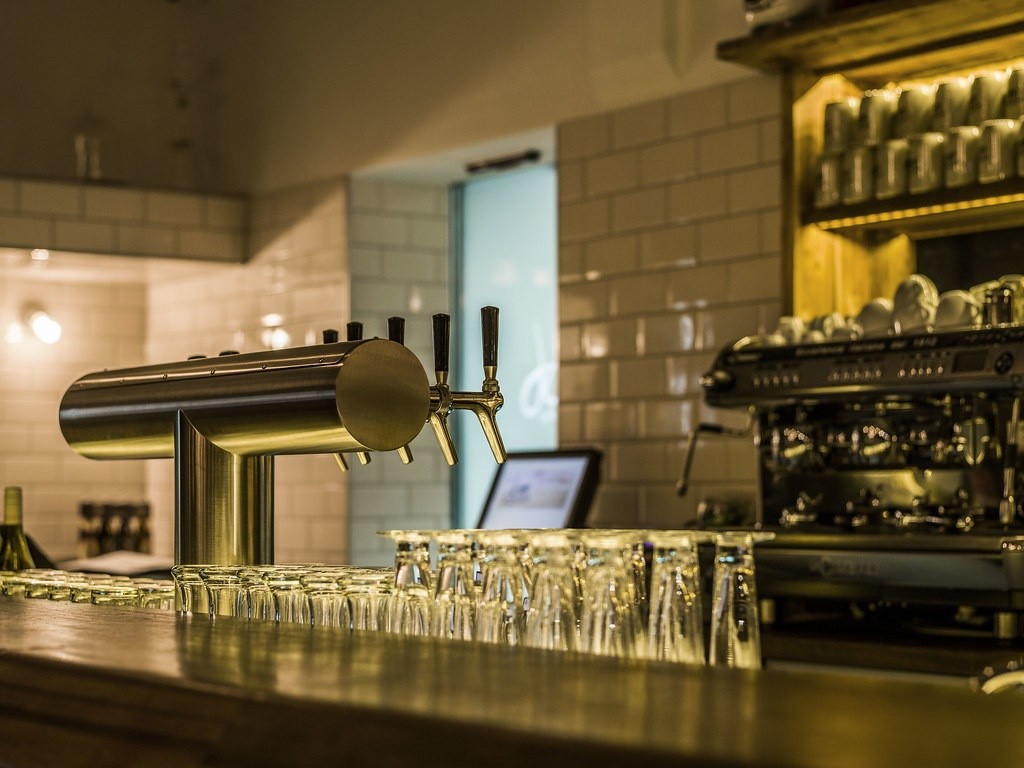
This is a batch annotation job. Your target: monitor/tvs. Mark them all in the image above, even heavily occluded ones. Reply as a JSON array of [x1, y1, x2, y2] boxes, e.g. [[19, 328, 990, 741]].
[[476, 449, 604, 535]]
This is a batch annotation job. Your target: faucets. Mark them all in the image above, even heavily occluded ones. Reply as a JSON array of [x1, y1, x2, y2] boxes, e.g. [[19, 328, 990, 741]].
[[323, 304, 507, 472]]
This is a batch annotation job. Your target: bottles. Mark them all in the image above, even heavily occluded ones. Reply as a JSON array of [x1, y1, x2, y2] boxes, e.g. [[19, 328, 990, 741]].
[[0, 486, 36, 570], [74, 99, 104, 178]]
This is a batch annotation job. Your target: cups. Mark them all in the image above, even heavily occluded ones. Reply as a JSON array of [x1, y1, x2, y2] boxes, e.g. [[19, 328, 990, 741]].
[[0, 567, 176, 611], [522, 528, 580, 652], [470, 530, 532, 649], [761, 274, 1024, 347], [430, 530, 477, 641], [575, 528, 648, 660], [375, 529, 436, 639], [169, 564, 396, 632], [643, 530, 776, 671]]
